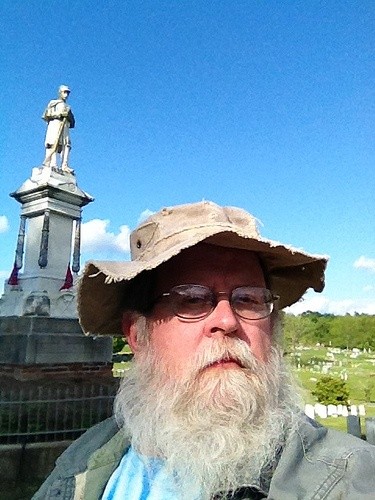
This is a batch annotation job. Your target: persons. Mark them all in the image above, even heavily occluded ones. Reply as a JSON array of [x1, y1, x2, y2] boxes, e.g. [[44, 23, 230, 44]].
[[41, 84, 77, 173], [28, 202, 375, 500]]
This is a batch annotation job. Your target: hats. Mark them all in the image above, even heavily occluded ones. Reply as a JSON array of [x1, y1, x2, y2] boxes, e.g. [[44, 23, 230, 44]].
[[76, 198, 328, 342]]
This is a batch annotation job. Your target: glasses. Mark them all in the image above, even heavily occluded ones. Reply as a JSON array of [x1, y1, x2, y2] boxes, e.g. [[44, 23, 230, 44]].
[[145, 283, 280, 321]]
